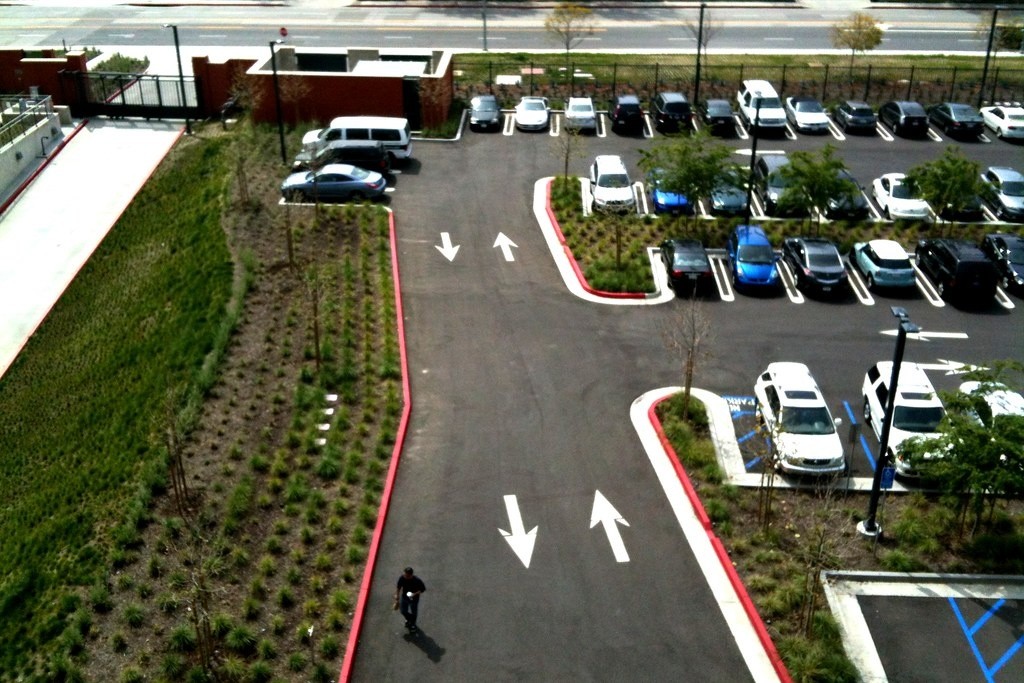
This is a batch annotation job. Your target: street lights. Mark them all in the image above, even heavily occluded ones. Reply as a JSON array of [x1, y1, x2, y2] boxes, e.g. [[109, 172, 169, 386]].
[[160, 24, 194, 136], [857, 303, 921, 538]]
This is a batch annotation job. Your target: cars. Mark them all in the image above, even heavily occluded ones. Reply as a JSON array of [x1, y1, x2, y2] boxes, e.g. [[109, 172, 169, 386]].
[[660, 238, 713, 292], [815, 166, 1024, 221], [785, 95, 829, 135], [834, 100, 1024, 140], [647, 169, 756, 215], [699, 99, 736, 137], [514, 96, 551, 131], [785, 240, 849, 298], [280, 163, 386, 203]]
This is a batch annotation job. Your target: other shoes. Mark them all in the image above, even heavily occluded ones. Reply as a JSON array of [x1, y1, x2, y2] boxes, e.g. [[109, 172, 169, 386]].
[[405, 620, 413, 626]]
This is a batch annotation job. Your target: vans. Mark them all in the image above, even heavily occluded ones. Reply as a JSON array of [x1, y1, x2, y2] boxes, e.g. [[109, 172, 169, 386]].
[[650, 93, 694, 133], [301, 116, 413, 164], [981, 233, 1024, 292]]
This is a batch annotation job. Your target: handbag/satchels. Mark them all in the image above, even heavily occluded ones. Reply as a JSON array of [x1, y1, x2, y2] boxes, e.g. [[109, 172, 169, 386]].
[[393, 599, 399, 611]]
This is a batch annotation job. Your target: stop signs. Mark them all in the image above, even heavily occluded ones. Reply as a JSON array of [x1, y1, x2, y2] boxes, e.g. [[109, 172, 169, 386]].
[[280, 27, 289, 41]]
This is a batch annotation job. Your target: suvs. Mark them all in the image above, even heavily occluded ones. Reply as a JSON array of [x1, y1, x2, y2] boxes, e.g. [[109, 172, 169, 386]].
[[469, 95, 500, 131], [736, 79, 787, 134], [726, 226, 782, 294], [758, 154, 808, 216], [916, 235, 998, 305], [290, 140, 388, 175], [588, 155, 636, 213], [608, 95, 645, 130], [754, 361, 1024, 488], [853, 238, 912, 292], [561, 96, 597, 130]]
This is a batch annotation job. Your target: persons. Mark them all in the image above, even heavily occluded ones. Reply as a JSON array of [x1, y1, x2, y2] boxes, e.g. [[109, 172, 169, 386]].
[[395, 567, 425, 629]]
